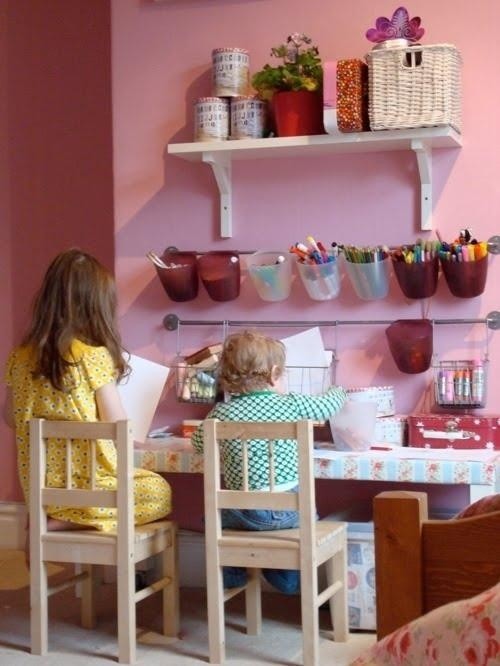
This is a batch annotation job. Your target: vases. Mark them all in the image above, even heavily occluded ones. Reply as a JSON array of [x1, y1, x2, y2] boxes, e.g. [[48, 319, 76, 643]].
[[268, 90, 321, 137]]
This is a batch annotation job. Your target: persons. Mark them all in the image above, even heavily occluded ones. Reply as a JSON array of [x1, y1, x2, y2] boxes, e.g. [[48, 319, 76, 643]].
[[189, 327, 346, 597], [4, 249, 173, 596]]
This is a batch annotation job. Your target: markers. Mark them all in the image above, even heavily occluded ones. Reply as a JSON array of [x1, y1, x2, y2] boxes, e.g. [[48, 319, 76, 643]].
[[440, 357, 483, 404], [228, 256, 237, 266]]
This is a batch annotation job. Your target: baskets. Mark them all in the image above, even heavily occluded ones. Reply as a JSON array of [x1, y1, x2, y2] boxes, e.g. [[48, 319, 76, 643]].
[[169, 356, 219, 404], [364, 44, 462, 134], [282, 349, 338, 429], [431, 352, 486, 408]]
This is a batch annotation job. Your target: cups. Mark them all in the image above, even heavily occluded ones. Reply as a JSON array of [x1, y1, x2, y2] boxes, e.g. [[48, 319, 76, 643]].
[[244, 249, 293, 302], [154, 248, 195, 302], [295, 257, 342, 302], [198, 249, 240, 302], [391, 258, 438, 299], [441, 254, 488, 298], [386, 319, 433, 374], [343, 257, 389, 299]]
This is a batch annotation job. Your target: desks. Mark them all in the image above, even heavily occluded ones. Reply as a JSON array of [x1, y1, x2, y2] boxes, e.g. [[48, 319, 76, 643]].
[[134, 436, 500, 505]]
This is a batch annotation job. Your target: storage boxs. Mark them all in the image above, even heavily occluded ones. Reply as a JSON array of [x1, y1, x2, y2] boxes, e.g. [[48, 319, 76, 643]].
[[321, 504, 462, 630], [408, 416, 500, 449]]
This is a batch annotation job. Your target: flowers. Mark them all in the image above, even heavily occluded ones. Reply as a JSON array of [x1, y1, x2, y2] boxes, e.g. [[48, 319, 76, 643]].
[[252, 32, 323, 100]]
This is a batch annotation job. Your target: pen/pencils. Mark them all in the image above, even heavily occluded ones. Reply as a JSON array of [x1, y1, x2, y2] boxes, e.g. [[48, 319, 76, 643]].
[[436, 227, 487, 262], [252, 256, 285, 289], [389, 238, 437, 263], [147, 250, 189, 269], [370, 446, 392, 451], [343, 245, 389, 299], [289, 236, 338, 299]]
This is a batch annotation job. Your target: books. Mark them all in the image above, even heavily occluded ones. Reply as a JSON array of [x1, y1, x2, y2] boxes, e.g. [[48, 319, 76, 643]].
[[185, 342, 224, 365], [186, 354, 220, 379]]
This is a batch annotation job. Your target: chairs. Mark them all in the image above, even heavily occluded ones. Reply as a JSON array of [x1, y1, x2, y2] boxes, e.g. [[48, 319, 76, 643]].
[[204, 418, 349, 666], [373, 491, 500, 642], [30, 417, 180, 664]]
